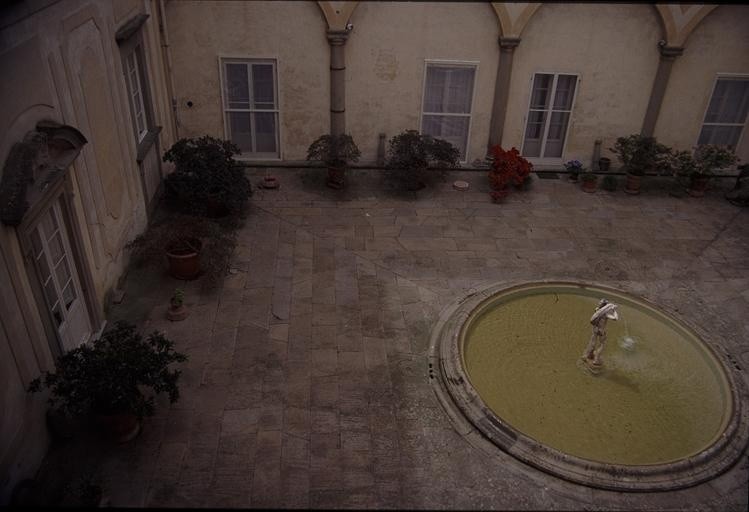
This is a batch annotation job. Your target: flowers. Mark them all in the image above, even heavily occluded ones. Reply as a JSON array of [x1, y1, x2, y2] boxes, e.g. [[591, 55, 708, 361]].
[[563, 159, 583, 172]]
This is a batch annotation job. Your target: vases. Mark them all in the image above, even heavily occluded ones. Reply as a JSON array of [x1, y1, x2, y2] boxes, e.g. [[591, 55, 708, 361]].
[[569, 167, 580, 181]]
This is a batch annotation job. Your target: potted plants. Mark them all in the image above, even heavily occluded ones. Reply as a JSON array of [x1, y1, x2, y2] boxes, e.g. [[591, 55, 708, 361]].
[[581, 132, 748, 207], [27, 322, 188, 447], [166, 290, 190, 323], [122, 211, 235, 279], [305, 130, 532, 204]]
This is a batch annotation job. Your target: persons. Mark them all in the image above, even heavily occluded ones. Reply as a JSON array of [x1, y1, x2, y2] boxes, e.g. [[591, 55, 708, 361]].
[[586, 299, 618, 364]]
